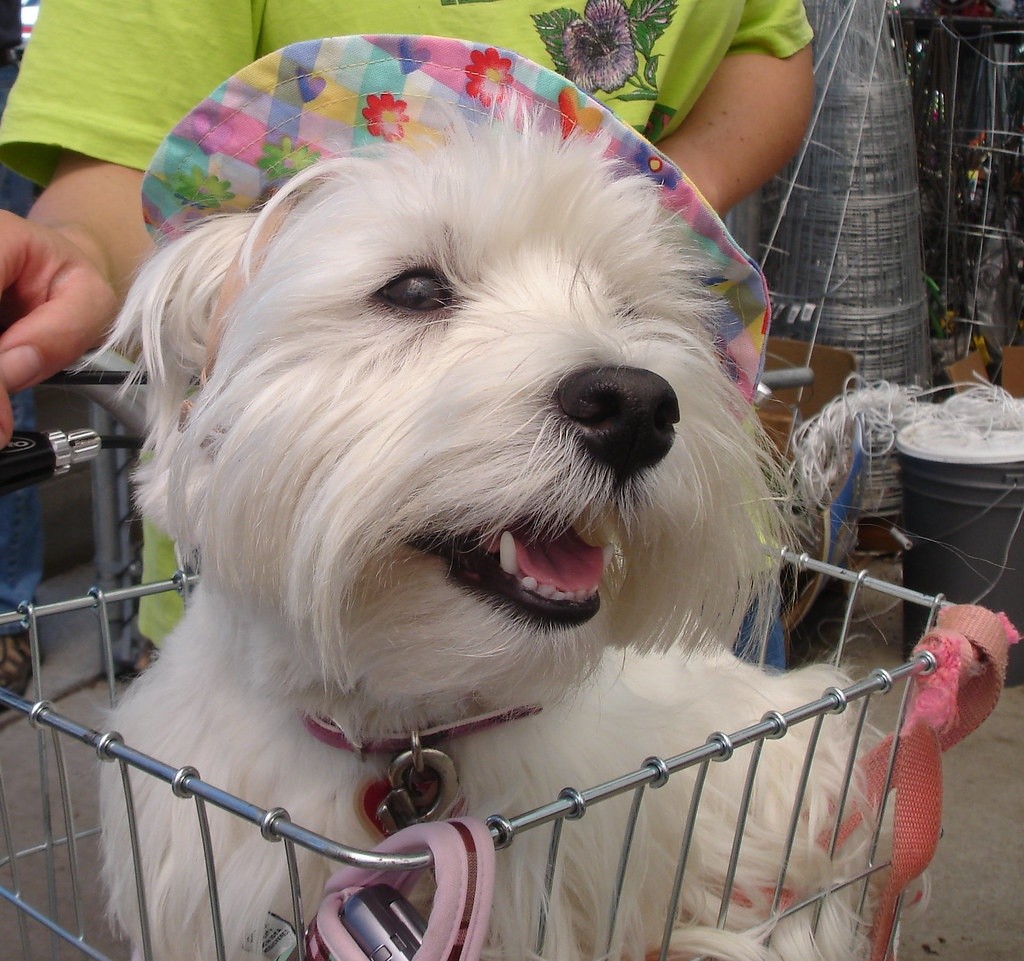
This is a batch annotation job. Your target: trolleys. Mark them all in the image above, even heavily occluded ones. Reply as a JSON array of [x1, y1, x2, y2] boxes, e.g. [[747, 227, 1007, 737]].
[[1, 347, 1019, 960]]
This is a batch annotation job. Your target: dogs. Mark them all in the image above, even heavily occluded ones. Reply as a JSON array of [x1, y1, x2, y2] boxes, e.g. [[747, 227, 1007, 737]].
[[99, 102, 871, 961]]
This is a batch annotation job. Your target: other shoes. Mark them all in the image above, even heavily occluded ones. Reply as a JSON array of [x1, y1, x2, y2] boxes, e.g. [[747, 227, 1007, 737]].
[[0, 628, 32, 711]]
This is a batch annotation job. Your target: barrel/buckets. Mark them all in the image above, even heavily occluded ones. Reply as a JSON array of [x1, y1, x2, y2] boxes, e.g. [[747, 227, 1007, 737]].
[[896, 419, 1023, 683]]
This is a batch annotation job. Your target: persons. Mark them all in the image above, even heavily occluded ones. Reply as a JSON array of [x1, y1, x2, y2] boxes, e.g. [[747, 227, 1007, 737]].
[[0, 0, 816, 670], [0, 64, 46, 713]]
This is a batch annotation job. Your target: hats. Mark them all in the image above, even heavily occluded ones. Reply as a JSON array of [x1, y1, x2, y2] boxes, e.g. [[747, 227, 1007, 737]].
[[144, 34, 771, 423]]
